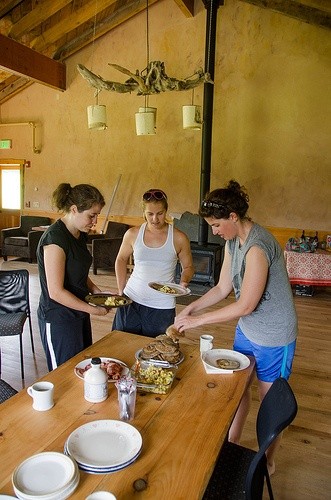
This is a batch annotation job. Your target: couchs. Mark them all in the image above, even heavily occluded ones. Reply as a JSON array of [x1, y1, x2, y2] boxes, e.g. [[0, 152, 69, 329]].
[[0, 215, 51, 264], [86, 221, 132, 275]]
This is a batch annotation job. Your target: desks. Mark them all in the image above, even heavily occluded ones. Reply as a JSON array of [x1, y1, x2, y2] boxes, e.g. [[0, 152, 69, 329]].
[[31, 225, 50, 231], [283, 247, 331, 295], [0, 330, 257, 500]]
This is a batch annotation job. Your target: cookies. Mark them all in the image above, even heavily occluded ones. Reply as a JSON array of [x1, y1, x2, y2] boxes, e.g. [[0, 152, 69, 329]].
[[140, 335, 182, 363]]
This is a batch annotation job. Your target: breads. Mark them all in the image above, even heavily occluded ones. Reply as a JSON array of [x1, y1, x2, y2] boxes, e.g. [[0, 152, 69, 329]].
[[217, 359, 240, 369], [166, 325, 185, 339]]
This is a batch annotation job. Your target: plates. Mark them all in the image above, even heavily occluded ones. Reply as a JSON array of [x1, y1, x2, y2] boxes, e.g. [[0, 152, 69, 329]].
[[84, 293, 132, 307], [201, 348, 250, 371], [74, 356, 130, 383], [147, 281, 191, 297], [63, 419, 144, 475], [134, 347, 186, 365], [0, 451, 81, 500]]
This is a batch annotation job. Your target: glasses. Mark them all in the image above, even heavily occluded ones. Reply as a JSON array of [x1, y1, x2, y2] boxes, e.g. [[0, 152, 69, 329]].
[[201, 200, 224, 209], [142, 191, 167, 201]]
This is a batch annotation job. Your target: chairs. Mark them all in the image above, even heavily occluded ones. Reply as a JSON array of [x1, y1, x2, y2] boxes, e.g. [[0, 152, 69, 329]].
[[201, 377, 297, 500], [0, 269, 35, 380], [0, 344, 18, 404]]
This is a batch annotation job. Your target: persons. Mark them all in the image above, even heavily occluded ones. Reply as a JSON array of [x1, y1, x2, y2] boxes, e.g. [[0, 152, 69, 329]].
[[175, 178, 298, 482], [112, 189, 193, 339], [36, 183, 110, 372]]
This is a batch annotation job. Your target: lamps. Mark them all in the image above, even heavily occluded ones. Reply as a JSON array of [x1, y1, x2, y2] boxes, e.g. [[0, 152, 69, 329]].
[[75, 0, 215, 135]]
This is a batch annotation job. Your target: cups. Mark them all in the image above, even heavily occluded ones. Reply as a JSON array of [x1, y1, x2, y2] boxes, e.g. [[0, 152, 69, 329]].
[[118, 388, 137, 422], [200, 334, 214, 352], [85, 490, 117, 500], [26, 381, 55, 411]]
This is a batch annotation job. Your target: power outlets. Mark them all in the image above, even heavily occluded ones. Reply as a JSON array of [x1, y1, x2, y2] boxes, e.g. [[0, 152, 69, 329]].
[[25, 201, 30, 207]]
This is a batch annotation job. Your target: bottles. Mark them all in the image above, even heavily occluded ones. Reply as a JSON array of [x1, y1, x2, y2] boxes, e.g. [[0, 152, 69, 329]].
[[84, 357, 109, 403], [300, 230, 306, 242], [313, 231, 319, 248]]
